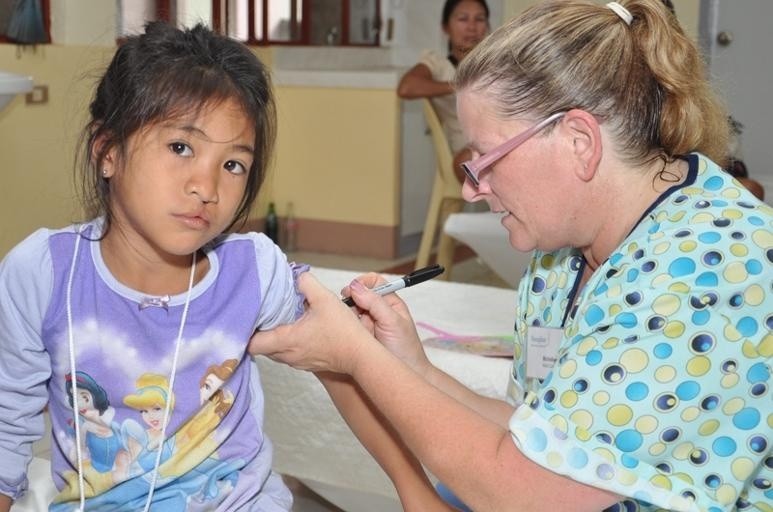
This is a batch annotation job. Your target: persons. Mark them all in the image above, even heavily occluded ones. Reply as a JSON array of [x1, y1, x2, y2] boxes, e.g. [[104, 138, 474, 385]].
[[397, 0, 491, 181], [249, 0, 771, 512], [0, 13, 462, 512]]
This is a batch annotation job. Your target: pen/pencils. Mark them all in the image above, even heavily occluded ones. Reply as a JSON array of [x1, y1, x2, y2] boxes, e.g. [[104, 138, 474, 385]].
[[341, 263, 445, 308]]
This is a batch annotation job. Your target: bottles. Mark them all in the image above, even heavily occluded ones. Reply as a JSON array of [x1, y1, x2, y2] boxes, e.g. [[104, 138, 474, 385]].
[[263, 202, 279, 244], [282, 200, 299, 252]]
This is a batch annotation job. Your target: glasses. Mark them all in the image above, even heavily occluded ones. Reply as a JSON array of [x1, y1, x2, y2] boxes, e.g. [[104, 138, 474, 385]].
[[460, 111, 605, 188]]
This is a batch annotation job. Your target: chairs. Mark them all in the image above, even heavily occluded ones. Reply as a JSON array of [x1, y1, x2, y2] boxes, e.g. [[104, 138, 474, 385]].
[[413, 94, 463, 281]]
